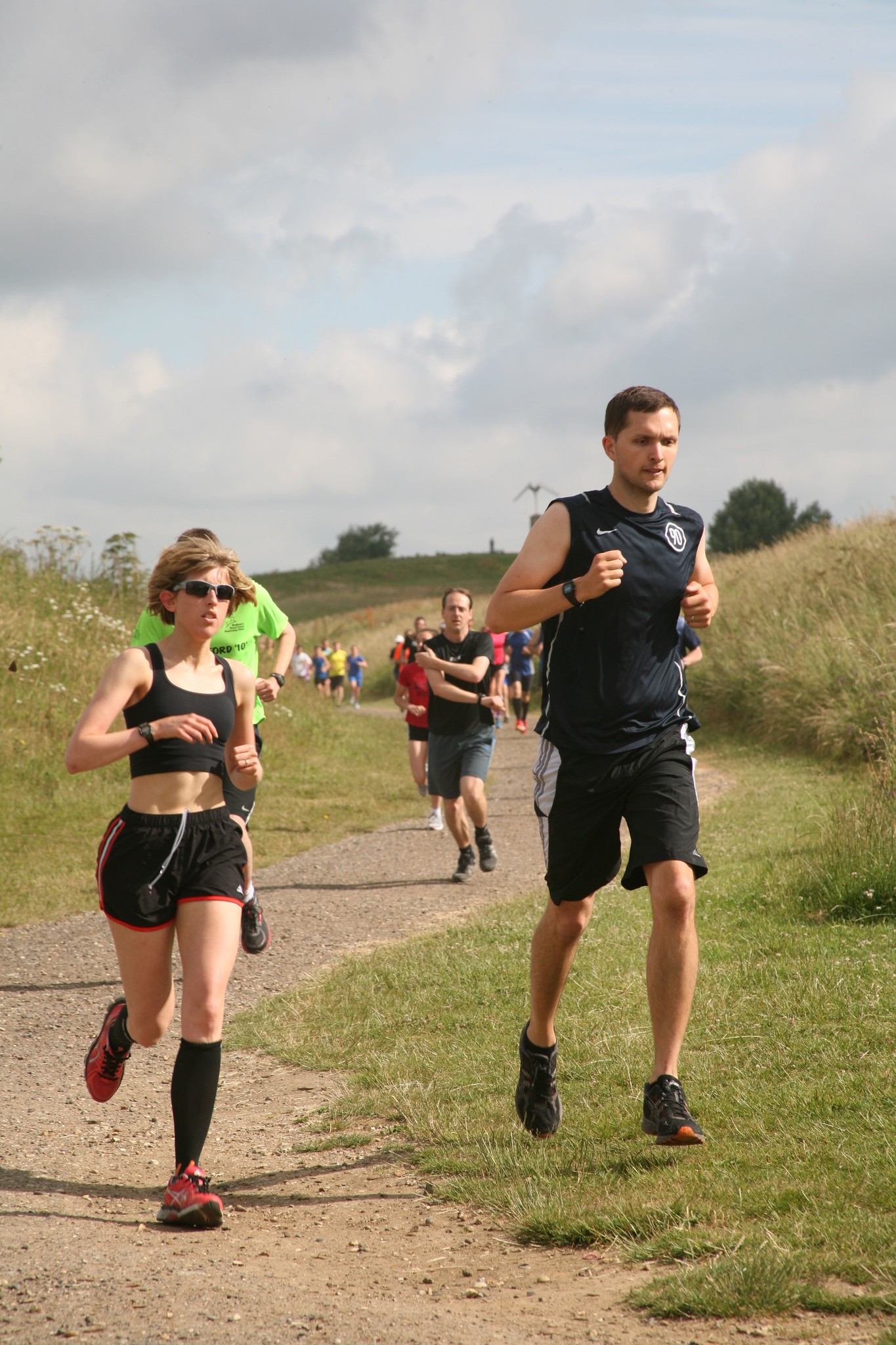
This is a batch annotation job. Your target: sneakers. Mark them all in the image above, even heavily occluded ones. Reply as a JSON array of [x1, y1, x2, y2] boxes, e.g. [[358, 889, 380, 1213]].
[[452, 853, 476, 882], [83, 995, 136, 1102], [415, 763, 428, 797], [514, 1018, 562, 1137], [493, 713, 528, 734], [156, 1165, 223, 1227], [641, 1074, 706, 1145], [475, 835, 498, 872], [428, 812, 444, 830], [240, 890, 271, 954]]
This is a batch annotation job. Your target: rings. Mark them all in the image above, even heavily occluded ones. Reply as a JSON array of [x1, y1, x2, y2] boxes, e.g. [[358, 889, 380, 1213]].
[[691, 615, 696, 623], [242, 758, 249, 767], [263, 694, 266, 699]]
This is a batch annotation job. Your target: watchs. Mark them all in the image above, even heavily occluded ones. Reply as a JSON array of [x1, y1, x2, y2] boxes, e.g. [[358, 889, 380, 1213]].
[[477, 691, 486, 707], [561, 578, 584, 608], [137, 721, 155, 745], [269, 673, 285, 687]]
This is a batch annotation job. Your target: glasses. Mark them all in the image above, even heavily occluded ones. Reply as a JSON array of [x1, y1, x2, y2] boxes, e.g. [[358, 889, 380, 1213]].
[[172, 579, 235, 600]]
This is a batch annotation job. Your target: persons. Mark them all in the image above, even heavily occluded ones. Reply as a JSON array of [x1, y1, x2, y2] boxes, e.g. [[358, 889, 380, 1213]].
[[413, 587, 506, 881], [65, 539, 265, 1232], [123, 529, 297, 956], [254, 603, 557, 739], [392, 629, 446, 831], [671, 616, 703, 669], [483, 384, 719, 1146]]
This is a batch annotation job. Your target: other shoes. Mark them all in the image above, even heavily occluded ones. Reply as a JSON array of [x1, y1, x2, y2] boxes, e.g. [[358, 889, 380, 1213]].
[[330, 695, 361, 710]]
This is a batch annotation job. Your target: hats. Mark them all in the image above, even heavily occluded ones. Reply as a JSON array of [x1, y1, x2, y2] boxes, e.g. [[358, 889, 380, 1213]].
[[394, 634, 404, 642]]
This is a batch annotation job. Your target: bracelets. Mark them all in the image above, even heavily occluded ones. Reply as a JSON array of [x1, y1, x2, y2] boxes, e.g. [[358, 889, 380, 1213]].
[[681, 658, 687, 670]]
